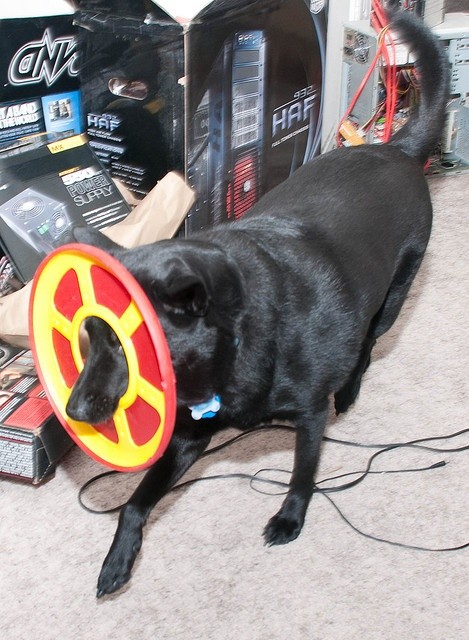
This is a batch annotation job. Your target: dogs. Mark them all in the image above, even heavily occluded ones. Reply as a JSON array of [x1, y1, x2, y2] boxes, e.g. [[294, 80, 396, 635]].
[[62, 2, 453, 603]]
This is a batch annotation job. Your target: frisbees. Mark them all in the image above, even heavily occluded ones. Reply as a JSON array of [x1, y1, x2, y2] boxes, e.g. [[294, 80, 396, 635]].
[[27, 242, 175, 474]]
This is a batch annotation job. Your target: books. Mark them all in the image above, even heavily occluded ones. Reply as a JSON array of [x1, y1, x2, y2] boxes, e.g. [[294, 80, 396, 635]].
[[0, 350, 76, 487]]
